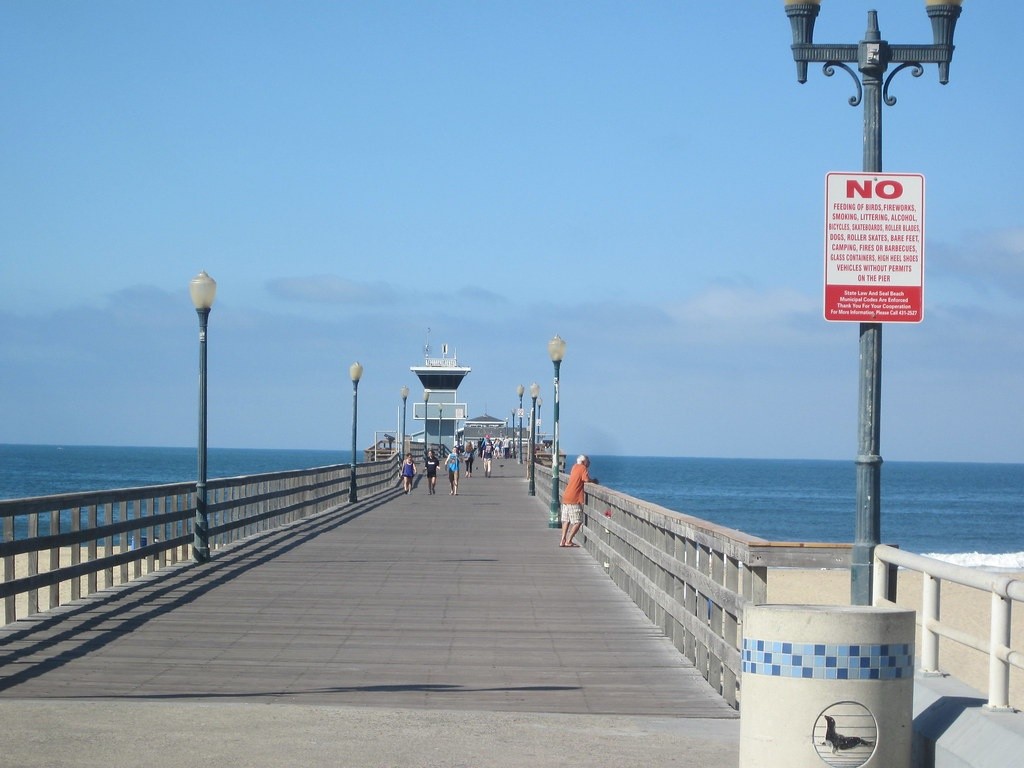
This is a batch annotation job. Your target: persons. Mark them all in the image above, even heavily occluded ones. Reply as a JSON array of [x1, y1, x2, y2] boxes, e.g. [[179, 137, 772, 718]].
[[457, 442, 474, 477], [401, 453, 417, 495], [477, 435, 510, 477], [425, 450, 439, 495], [445, 447, 463, 495], [560, 455, 599, 547]]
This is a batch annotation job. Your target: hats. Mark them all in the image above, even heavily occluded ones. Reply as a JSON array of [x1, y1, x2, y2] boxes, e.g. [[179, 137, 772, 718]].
[[486, 435, 490, 439]]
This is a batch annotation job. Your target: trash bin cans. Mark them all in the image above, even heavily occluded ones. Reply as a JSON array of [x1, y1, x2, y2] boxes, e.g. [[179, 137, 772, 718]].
[[737, 603, 915, 768]]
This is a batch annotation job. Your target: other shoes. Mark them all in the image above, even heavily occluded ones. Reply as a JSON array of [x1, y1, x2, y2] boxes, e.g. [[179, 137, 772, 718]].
[[449, 491, 454, 495], [408, 492, 412, 494], [454, 494, 457, 495], [428, 493, 431, 495], [404, 490, 407, 494], [432, 488, 435, 494]]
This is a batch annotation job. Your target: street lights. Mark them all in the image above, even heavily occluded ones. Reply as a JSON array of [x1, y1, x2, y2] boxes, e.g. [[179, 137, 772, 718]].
[[400, 385, 410, 463], [348, 360, 363, 503], [510, 408, 517, 459], [422, 391, 430, 463], [505, 417, 510, 438], [189, 269, 217, 563], [436, 402, 444, 459], [536, 396, 543, 444], [517, 384, 526, 464], [547, 334, 569, 528], [528, 381, 541, 496]]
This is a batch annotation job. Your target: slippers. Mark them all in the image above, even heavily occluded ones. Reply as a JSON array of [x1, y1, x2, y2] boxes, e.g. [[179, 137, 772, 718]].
[[559, 544, 564, 547], [564, 542, 580, 547]]
